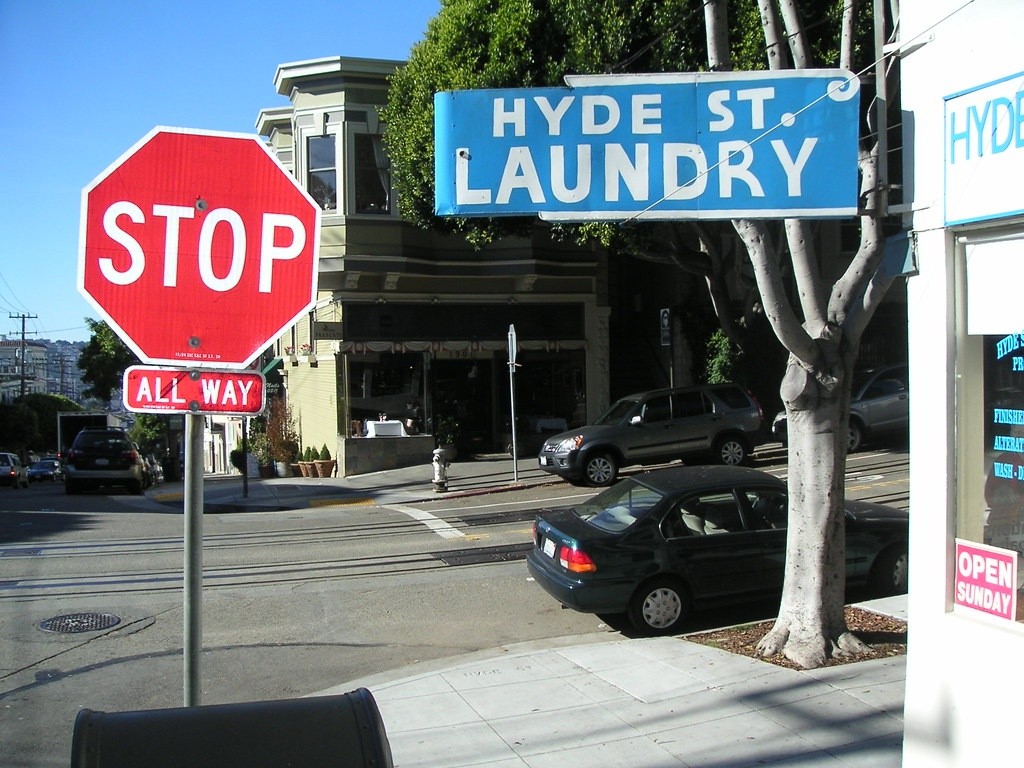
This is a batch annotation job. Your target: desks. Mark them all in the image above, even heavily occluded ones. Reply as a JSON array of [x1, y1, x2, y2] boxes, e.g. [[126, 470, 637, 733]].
[[363, 418, 403, 437]]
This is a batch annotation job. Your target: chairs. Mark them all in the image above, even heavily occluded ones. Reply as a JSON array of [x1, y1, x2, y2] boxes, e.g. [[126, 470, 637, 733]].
[[680, 499, 707, 537], [403, 417, 416, 435], [351, 419, 366, 437], [703, 508, 735, 536]]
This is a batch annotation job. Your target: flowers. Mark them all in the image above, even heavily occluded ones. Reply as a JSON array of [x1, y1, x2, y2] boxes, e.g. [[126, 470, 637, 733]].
[[300, 344, 312, 356], [284, 346, 295, 356]]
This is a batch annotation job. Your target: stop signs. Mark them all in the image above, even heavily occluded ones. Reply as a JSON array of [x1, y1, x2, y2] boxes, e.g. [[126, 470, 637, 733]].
[[78, 126, 321, 369]]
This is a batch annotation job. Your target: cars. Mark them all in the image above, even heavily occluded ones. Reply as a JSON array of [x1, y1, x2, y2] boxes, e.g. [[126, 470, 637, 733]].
[[768, 362, 908, 454], [22, 459, 61, 482], [526, 464, 907, 641]]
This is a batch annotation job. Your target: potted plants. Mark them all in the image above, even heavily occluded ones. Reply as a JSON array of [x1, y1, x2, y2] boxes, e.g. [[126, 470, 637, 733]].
[[298, 446, 312, 478], [229, 414, 276, 479], [313, 442, 337, 478], [303, 445, 320, 479], [264, 392, 302, 478], [289, 450, 304, 477], [433, 415, 461, 462]]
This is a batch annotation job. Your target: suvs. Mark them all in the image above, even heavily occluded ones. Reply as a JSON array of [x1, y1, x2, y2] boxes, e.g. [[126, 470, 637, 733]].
[[0, 452, 29, 488], [538, 378, 766, 488], [63, 427, 145, 496]]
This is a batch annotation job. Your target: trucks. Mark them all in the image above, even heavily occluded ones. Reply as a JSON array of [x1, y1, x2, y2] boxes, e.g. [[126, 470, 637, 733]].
[[54, 412, 123, 471]]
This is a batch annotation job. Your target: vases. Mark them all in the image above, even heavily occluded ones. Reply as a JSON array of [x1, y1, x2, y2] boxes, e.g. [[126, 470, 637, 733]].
[[285, 356, 295, 362], [300, 355, 312, 363]]
